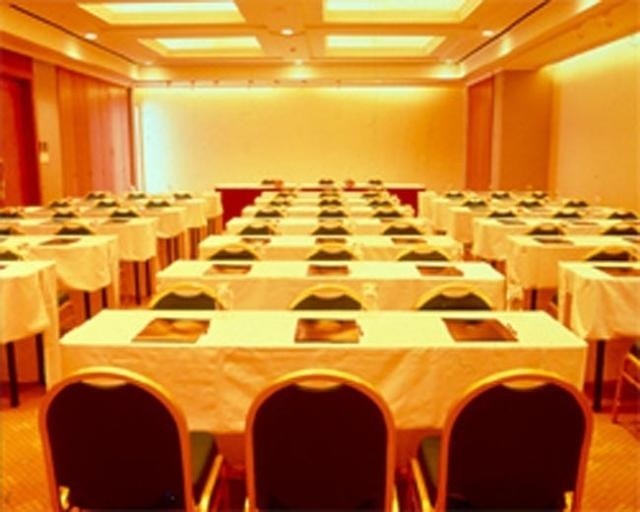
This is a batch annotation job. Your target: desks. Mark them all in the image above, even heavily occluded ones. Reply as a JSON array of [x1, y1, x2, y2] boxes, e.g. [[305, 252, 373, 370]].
[[55, 302, 591, 430], [222, 214, 427, 235], [213, 183, 428, 230], [149, 252, 506, 310], [196, 231, 462, 261]]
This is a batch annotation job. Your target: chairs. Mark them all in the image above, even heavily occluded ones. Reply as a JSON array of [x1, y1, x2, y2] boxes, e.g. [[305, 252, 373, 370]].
[[145, 284, 227, 310], [0, 179, 235, 409], [409, 367, 594, 511], [385, 220, 423, 235], [311, 219, 349, 234], [414, 279, 495, 309], [239, 223, 276, 234], [210, 245, 260, 262], [36, 367, 233, 509], [311, 242, 356, 260], [290, 281, 367, 314], [397, 244, 452, 263], [242, 370, 398, 511], [414, 187, 640, 432]]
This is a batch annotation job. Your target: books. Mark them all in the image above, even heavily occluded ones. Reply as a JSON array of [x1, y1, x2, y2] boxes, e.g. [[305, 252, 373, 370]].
[[201, 191, 296, 276], [364, 191, 464, 277], [1, 210, 82, 270], [308, 191, 352, 277], [128, 318, 209, 344], [595, 212, 639, 277], [294, 317, 357, 343], [441, 317, 517, 342], [110, 218, 129, 223], [446, 189, 597, 247]]
